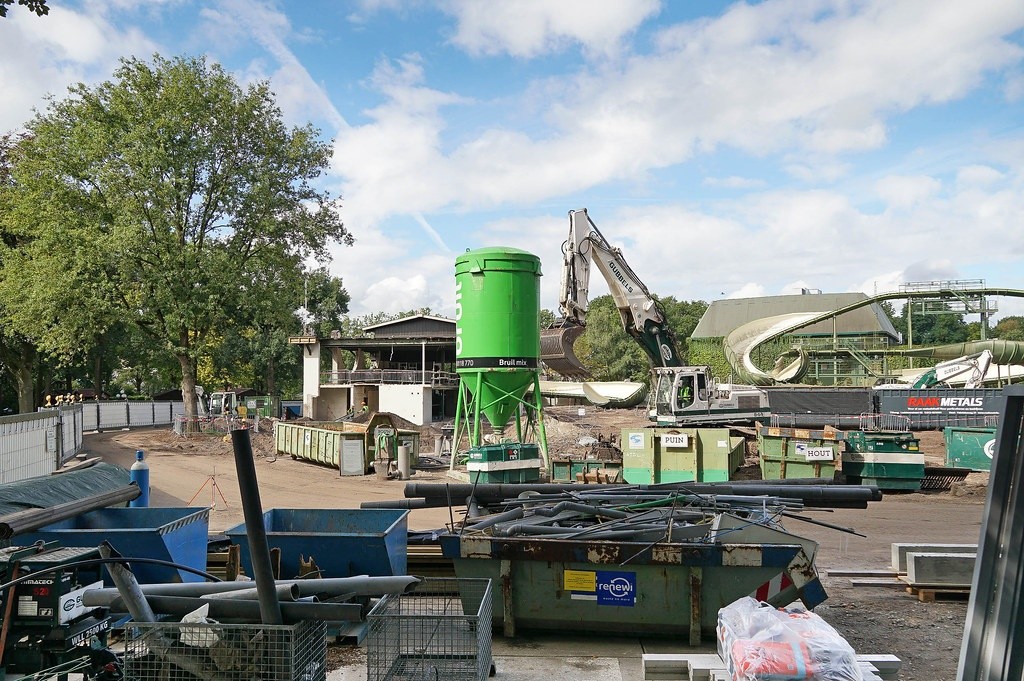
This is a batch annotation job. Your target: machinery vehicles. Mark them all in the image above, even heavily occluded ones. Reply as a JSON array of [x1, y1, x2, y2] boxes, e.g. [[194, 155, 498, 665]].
[[541, 208, 771, 430]]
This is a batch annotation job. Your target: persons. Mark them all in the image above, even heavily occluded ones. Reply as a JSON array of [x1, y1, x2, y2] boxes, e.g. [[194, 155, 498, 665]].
[[677, 382, 692, 410]]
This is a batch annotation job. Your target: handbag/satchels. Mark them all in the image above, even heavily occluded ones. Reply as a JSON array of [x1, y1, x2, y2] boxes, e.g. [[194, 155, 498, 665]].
[[715, 596, 863, 681]]
